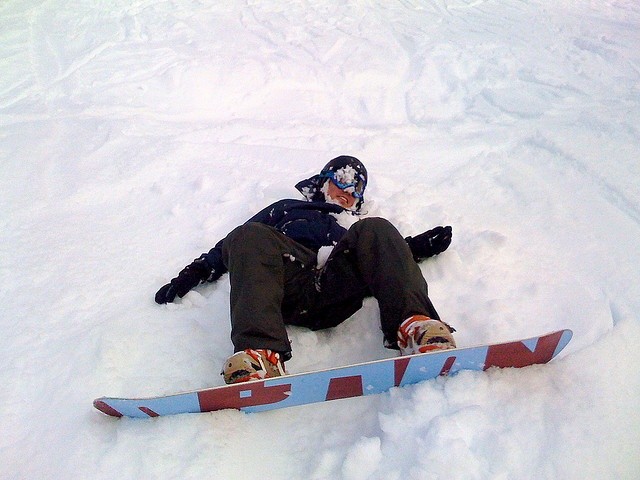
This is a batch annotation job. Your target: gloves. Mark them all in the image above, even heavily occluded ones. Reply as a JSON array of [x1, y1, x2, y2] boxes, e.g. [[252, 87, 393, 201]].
[[405, 226, 452, 262], [155, 253, 222, 304]]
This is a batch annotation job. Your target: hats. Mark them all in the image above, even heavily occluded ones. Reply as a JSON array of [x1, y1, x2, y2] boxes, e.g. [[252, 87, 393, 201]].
[[295, 156, 366, 211]]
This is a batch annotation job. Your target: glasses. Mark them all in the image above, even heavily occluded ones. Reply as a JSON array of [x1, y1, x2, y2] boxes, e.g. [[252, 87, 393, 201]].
[[320, 172, 366, 198]]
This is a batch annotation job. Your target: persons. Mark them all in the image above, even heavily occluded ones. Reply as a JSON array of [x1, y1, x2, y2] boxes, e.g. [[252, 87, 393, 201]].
[[155, 156, 455, 385]]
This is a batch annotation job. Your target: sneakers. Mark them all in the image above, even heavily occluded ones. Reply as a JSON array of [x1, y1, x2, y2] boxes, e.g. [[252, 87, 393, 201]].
[[223, 348, 285, 385], [398, 315, 457, 354]]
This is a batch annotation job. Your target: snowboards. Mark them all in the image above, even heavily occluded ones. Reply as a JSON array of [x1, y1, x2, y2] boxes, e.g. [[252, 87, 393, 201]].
[[92, 328, 572, 418]]
